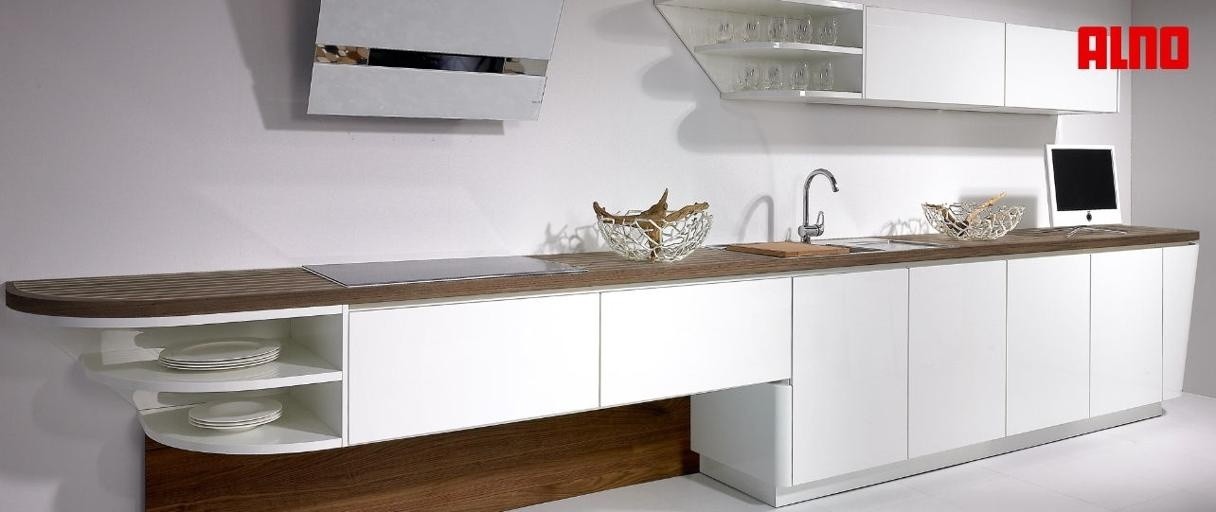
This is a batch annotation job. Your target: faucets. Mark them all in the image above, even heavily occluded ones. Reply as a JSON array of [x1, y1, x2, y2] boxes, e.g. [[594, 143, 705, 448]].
[[799, 168, 839, 244]]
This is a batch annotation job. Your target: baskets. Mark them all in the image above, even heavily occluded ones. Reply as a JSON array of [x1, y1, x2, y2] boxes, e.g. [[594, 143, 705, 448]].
[[921, 202, 1027, 241], [596, 209, 714, 263]]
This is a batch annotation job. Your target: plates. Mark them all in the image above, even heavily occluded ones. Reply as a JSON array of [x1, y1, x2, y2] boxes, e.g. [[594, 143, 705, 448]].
[[157, 337, 281, 371], [187, 395, 283, 434]]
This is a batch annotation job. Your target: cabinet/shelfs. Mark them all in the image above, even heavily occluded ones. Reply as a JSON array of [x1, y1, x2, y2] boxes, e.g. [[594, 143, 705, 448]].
[[687, 241, 1200, 507], [656, 1, 1121, 117], [116, 272, 791, 456]]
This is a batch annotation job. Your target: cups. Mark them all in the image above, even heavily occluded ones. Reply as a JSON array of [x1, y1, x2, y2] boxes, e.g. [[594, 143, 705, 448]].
[[712, 14, 841, 91]]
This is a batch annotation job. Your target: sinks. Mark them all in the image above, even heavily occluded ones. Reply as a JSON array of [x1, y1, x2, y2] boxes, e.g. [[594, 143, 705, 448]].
[[819, 240, 884, 255]]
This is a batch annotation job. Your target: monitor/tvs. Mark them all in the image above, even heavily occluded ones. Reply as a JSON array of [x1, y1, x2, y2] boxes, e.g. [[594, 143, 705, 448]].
[[1044, 143, 1126, 238]]
